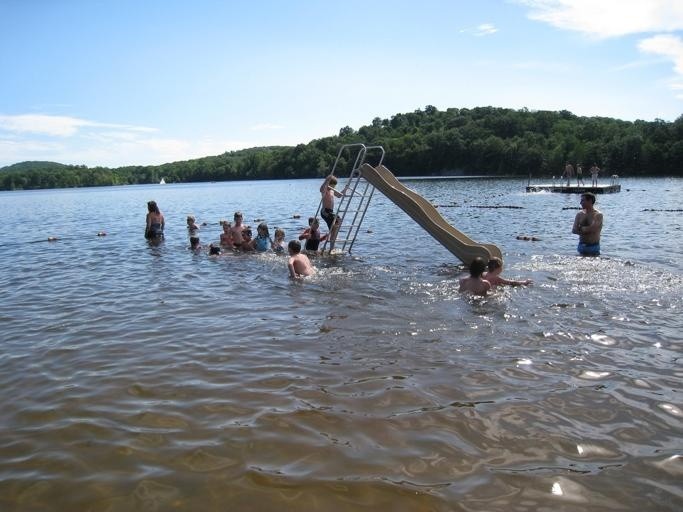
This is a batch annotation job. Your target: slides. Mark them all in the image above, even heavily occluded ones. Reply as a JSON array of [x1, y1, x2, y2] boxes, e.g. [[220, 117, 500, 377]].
[[360, 163, 503, 267]]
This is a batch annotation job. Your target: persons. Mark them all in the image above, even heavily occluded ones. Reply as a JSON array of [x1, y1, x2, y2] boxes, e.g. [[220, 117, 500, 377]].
[[572, 192, 603, 254], [144, 201, 165, 244], [187, 212, 328, 256], [482, 257, 533, 287], [459, 257, 491, 296], [562, 161, 600, 188], [320, 175, 347, 250], [288, 240, 313, 278]]
